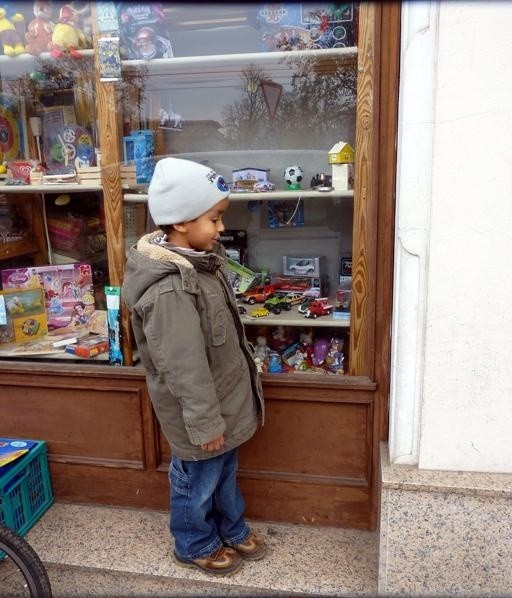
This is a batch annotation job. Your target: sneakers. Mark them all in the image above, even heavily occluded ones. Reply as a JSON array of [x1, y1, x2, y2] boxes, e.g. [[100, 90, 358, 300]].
[[228, 531, 267, 563], [170, 543, 242, 579]]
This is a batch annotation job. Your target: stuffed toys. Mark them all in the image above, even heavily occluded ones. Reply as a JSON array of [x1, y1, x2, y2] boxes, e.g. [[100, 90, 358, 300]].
[[48, 0, 91, 60], [1, 0, 27, 56], [26, 0, 60, 55]]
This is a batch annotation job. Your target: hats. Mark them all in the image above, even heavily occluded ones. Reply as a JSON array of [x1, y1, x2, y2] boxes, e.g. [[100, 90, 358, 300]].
[[146, 155, 233, 227]]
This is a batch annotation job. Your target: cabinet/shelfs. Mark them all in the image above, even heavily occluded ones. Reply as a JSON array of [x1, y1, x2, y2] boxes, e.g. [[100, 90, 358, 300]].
[[0, 0, 402, 532]]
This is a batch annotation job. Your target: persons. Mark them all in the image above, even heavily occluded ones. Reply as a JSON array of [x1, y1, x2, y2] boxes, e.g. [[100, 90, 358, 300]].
[[119, 156, 269, 575]]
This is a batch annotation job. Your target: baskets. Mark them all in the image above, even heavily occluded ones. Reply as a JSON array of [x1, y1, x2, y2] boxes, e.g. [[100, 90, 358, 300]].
[[0, 437, 57, 564]]
